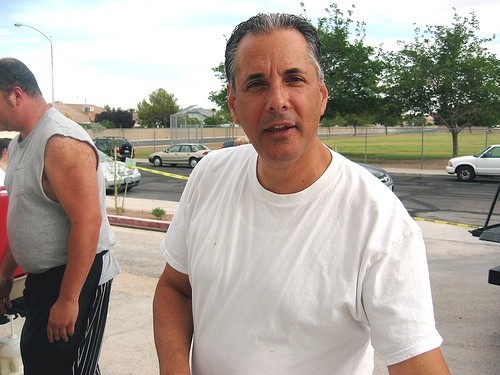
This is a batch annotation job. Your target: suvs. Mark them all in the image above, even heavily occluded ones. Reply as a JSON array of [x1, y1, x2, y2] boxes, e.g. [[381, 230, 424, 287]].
[[91, 137, 134, 162]]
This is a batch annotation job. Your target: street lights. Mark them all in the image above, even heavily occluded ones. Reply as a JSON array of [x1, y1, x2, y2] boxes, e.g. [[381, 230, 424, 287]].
[[13, 22, 55, 108]]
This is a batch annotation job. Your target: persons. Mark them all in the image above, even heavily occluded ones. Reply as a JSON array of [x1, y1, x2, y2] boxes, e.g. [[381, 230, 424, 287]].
[[152, 14, 453, 375], [234, 136, 249, 146], [0, 58, 123, 374]]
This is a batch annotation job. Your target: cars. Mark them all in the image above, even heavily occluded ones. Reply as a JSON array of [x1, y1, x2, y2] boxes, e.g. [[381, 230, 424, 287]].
[[99, 150, 141, 194], [148, 143, 212, 168], [324, 144, 394, 194], [446, 144, 500, 182]]
